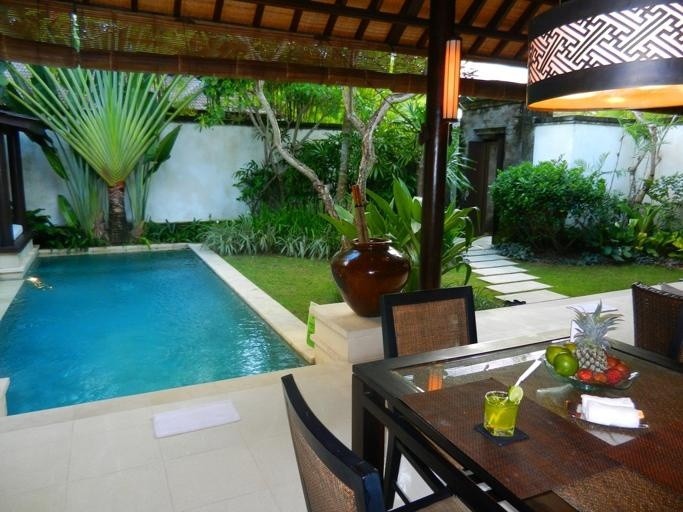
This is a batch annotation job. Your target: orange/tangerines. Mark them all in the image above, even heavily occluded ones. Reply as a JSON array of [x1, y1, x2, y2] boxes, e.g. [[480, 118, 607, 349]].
[[546, 341, 580, 377]]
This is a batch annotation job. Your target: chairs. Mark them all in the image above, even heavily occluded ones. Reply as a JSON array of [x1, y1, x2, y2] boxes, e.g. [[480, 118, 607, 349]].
[[631, 282, 683, 361], [379, 285, 484, 509], [280, 373, 468, 510]]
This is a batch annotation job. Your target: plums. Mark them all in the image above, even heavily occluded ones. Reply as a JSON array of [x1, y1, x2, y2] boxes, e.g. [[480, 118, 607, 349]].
[[578, 356, 630, 384]]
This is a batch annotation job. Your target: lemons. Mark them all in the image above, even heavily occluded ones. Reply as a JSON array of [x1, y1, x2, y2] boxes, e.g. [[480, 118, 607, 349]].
[[508, 385, 523, 404]]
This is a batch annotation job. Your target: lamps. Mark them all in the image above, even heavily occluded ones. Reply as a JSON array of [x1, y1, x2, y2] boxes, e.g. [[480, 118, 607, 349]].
[[442, 36, 462, 123], [526, 0, 683, 112]]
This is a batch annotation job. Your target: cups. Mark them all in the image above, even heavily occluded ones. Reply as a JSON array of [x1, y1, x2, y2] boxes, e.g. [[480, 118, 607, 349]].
[[483, 390, 520, 438]]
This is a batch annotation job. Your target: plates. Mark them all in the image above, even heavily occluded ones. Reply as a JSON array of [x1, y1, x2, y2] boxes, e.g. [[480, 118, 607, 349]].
[[541, 352, 641, 394]]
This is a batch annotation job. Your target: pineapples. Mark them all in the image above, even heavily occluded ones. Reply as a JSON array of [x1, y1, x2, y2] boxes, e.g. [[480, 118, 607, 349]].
[[566, 299, 625, 373]]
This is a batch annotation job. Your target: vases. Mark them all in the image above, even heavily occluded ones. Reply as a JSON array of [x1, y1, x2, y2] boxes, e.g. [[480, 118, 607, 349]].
[[331, 238, 410, 317]]
